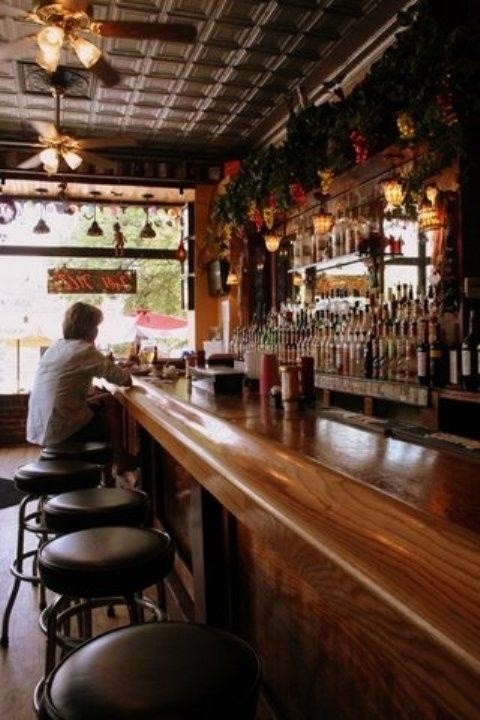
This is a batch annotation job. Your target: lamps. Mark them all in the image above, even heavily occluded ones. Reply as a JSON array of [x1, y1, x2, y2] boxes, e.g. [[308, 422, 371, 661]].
[[33, 188, 50, 233], [34, 16, 102, 70], [225, 237, 241, 287], [312, 192, 334, 235], [139, 193, 156, 237], [32, 142, 83, 173], [381, 153, 408, 207], [88, 191, 103, 236], [417, 186, 440, 232], [262, 221, 283, 253]]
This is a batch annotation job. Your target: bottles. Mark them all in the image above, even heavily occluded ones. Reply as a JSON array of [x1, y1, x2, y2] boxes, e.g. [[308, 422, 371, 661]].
[[296, 357, 314, 397], [475, 330, 480, 392], [275, 357, 296, 364], [150, 346, 159, 366], [292, 218, 370, 270], [314, 284, 447, 372], [260, 396, 272, 425], [268, 294, 315, 326], [259, 353, 275, 396], [461, 310, 475, 390], [278, 366, 296, 401], [282, 402, 300, 447], [268, 385, 281, 407], [231, 325, 259, 361], [449, 322, 461, 384], [259, 325, 314, 357]]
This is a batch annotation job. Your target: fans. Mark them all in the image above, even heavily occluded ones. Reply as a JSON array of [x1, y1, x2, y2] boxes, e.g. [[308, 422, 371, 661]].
[[0, 0, 197, 87], [0, 83, 139, 175]]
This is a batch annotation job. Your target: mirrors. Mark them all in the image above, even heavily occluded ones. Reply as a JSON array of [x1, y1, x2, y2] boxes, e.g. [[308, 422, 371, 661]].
[[275, 193, 433, 315]]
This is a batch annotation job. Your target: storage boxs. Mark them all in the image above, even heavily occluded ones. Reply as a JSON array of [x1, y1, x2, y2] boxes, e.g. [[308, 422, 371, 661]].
[[191, 365, 245, 396]]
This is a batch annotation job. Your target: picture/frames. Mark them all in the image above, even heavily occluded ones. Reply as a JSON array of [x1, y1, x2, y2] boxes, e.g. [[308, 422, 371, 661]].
[[180, 239, 194, 275], [179, 201, 194, 239], [181, 276, 194, 312]]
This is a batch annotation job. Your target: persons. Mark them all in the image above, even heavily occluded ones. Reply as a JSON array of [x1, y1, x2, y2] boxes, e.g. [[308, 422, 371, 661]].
[[25, 302, 141, 488]]
